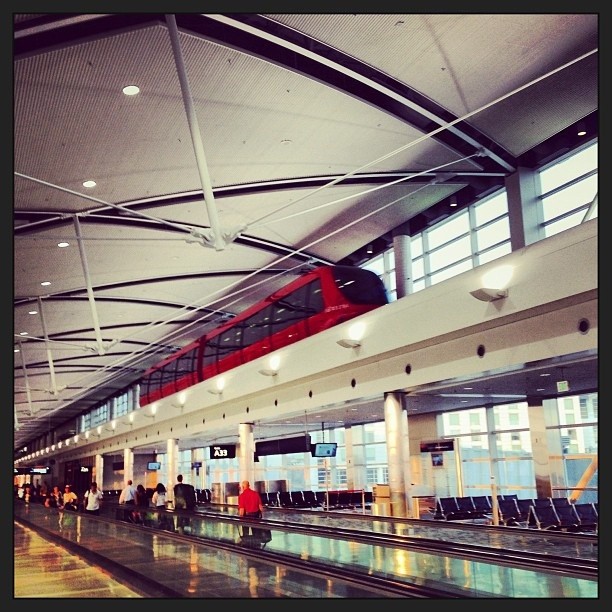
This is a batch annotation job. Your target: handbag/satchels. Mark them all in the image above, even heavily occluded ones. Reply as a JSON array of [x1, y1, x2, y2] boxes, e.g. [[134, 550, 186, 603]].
[[64, 500, 80, 512], [258, 519, 272, 542]]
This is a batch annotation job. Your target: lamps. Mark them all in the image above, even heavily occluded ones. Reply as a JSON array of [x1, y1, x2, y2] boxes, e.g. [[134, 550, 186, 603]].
[[258, 370, 278, 376], [467, 288, 508, 302], [335, 339, 361, 349], [172, 404, 184, 409], [207, 390, 222, 396]]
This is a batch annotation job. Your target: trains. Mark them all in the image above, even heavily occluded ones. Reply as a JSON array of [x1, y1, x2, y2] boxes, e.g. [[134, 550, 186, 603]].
[[140, 266, 389, 407]]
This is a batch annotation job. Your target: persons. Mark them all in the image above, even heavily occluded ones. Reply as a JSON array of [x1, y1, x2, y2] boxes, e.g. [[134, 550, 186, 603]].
[[174, 475, 195, 508], [35, 479, 39, 489], [134, 485, 149, 507], [119, 479, 136, 506], [239, 481, 263, 518], [152, 483, 167, 509], [44, 486, 63, 509], [62, 484, 77, 512], [82, 482, 103, 516], [44, 481, 50, 494]]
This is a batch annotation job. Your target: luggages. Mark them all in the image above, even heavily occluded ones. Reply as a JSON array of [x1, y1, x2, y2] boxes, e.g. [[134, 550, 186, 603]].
[[128, 508, 145, 525], [236, 526, 262, 548]]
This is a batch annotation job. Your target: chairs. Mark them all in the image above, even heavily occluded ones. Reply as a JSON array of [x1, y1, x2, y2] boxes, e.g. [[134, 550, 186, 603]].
[[551, 498, 571, 506], [314, 492, 327, 507], [534, 497, 552, 506], [427, 498, 440, 519], [267, 492, 279, 507], [471, 496, 491, 515], [324, 490, 372, 511], [527, 504, 576, 530], [278, 492, 293, 507], [260, 493, 269, 507], [290, 491, 307, 507], [482, 500, 515, 520], [553, 503, 596, 529], [488, 495, 504, 510], [438, 497, 470, 521], [498, 499, 521, 527], [517, 499, 534, 521], [593, 503, 598, 512], [456, 497, 476, 516], [502, 495, 518, 501], [303, 490, 317, 506]]
